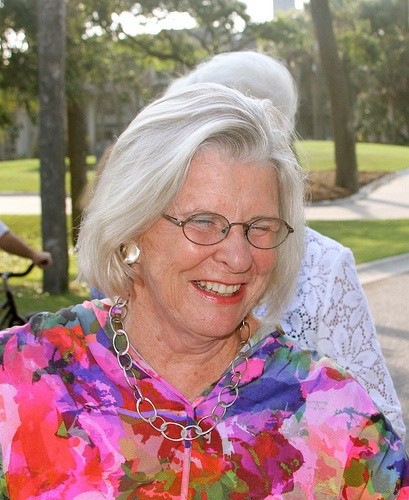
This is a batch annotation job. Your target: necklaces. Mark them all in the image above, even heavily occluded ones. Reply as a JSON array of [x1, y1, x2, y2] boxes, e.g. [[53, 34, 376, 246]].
[[109, 294, 252, 441]]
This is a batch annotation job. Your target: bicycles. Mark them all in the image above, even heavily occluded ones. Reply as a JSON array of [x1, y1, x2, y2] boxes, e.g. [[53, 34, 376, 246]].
[[0, 259, 49, 330]]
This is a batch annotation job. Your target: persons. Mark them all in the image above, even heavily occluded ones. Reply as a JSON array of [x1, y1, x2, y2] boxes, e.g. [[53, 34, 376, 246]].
[[162, 49, 406, 443], [0, 81, 409, 500], [0, 220, 52, 269]]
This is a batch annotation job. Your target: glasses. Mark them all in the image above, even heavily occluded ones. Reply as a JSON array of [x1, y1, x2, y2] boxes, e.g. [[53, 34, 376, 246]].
[[161, 211, 294, 250]]
